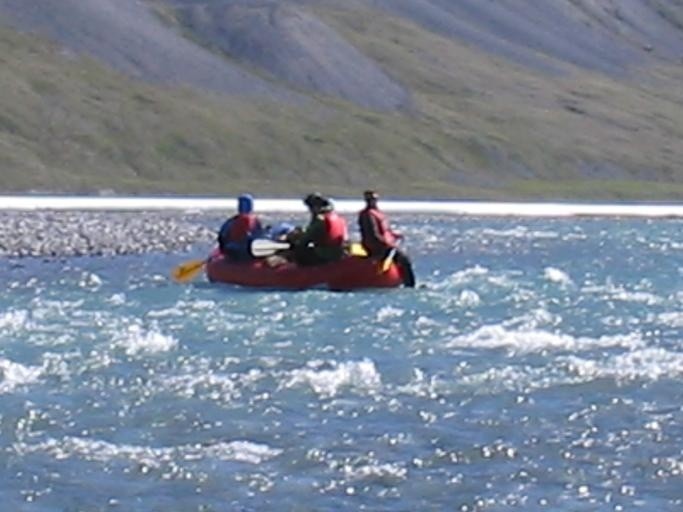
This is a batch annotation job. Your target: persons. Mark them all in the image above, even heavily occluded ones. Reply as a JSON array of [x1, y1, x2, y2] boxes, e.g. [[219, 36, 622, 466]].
[[293, 226, 302, 234], [357, 190, 414, 287], [276, 234, 288, 243], [263, 224, 272, 239], [217, 194, 267, 263], [287, 192, 349, 266]]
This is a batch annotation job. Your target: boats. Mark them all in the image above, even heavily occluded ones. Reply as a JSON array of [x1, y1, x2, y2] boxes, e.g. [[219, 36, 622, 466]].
[[200, 238, 403, 291]]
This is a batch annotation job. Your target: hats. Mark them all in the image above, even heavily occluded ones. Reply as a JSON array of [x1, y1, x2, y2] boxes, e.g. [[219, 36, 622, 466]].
[[238, 193, 253, 213], [363, 190, 380, 199], [304, 192, 333, 214]]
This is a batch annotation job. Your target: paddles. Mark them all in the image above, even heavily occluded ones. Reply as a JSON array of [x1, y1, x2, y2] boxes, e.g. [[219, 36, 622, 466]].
[[342, 243, 369, 257], [376, 238, 402, 274], [250, 237, 291, 257], [171, 250, 224, 282]]
